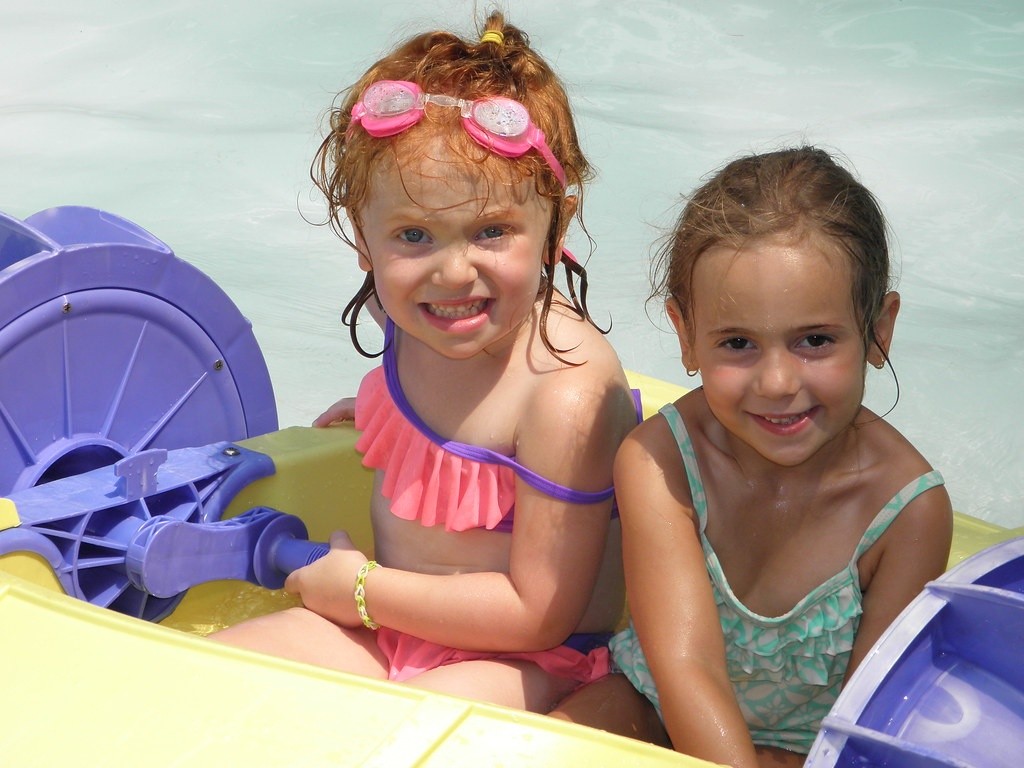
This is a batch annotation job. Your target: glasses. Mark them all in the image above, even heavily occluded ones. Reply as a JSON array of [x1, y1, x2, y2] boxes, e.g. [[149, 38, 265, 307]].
[[343, 80, 568, 191]]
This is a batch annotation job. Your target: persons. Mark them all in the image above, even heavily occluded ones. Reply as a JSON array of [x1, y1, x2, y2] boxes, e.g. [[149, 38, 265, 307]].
[[205, 10, 637, 716], [544, 146, 954, 768]]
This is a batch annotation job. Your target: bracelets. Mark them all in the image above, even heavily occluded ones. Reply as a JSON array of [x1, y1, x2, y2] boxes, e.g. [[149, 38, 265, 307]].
[[354, 560, 380, 632]]
